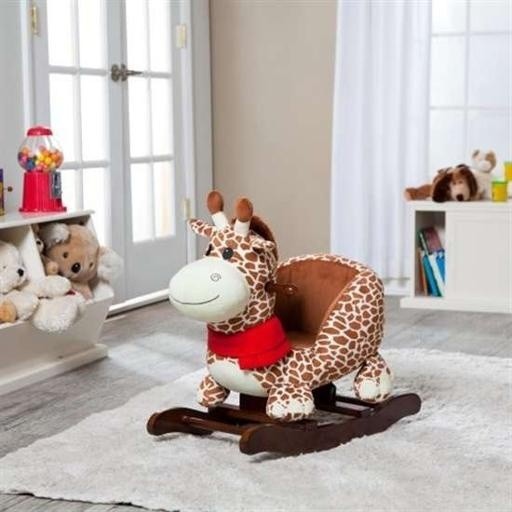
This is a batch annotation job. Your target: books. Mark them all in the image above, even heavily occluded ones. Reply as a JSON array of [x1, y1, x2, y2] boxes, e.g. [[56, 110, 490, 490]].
[[415, 223, 445, 299]]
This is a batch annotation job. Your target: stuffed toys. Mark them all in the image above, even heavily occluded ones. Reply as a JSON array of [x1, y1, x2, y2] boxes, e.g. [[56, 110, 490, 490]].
[[0, 238, 89, 333], [39, 221, 122, 299], [145, 189, 422, 454], [31, 223, 60, 276], [468, 150, 497, 202], [402, 164, 486, 204]]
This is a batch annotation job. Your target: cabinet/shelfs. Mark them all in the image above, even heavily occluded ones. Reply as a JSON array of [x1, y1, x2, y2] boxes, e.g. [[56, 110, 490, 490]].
[[399, 200, 512, 315], [0, 210, 115, 398]]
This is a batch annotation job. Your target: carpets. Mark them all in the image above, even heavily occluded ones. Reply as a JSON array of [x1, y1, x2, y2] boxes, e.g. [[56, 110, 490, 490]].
[[0, 344, 511, 512]]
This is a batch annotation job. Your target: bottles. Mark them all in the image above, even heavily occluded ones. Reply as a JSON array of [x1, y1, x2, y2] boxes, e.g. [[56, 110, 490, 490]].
[[492, 181, 507, 201]]
